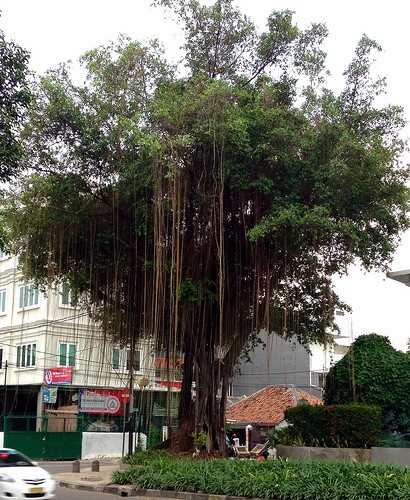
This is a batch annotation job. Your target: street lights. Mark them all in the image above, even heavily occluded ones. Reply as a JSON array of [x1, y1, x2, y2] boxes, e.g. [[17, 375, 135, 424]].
[[135, 377, 149, 453]]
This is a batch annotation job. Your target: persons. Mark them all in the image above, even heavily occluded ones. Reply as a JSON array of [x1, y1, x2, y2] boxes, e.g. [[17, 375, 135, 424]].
[[232, 433, 240, 446]]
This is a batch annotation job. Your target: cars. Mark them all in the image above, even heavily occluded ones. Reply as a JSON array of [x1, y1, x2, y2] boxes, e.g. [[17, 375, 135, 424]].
[[0, 447, 56, 500]]
